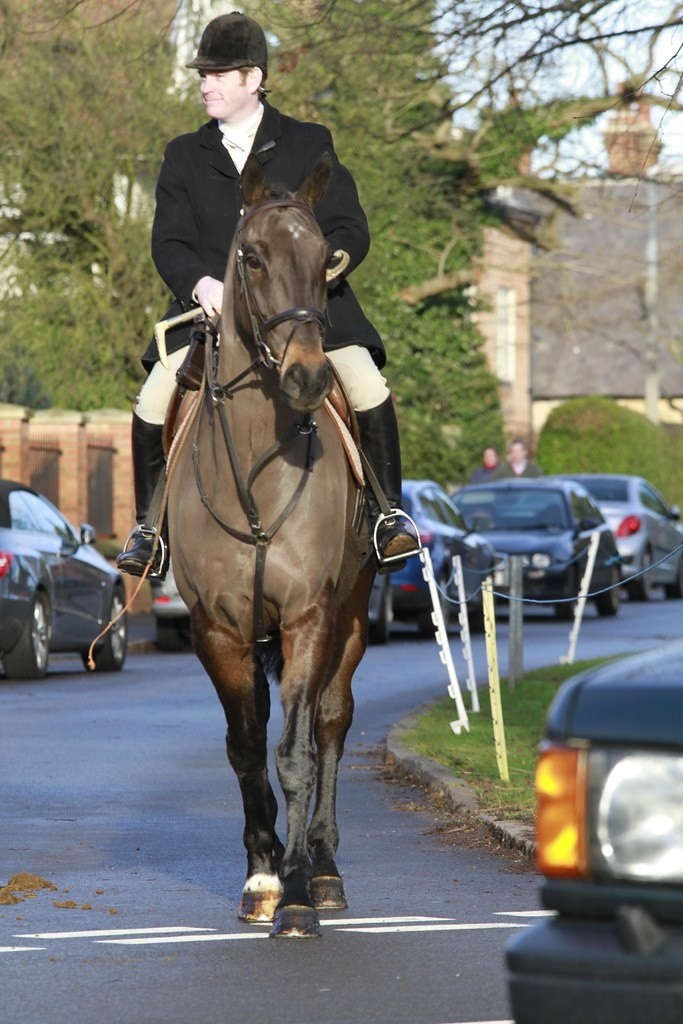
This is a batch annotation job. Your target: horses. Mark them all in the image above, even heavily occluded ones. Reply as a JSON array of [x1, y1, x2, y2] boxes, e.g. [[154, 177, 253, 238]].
[[166, 149, 386, 940]]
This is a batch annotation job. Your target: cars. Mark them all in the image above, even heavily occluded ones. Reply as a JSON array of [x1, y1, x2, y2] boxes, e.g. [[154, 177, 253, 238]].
[[0, 478, 130, 676], [384, 480, 496, 628], [148, 543, 396, 651], [550, 475, 683, 597], [503, 622, 682, 1024], [444, 477, 623, 621]]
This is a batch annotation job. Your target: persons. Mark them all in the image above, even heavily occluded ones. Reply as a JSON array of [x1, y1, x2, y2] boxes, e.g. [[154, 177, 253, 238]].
[[115, 10, 418, 582], [499, 440, 541, 479], [468, 446, 505, 484]]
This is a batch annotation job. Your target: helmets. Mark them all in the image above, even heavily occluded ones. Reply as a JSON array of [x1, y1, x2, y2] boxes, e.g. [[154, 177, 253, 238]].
[[185, 10, 268, 80]]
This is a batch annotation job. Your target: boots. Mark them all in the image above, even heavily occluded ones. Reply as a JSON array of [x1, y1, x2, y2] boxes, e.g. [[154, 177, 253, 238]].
[[116, 401, 170, 581], [354, 387, 419, 575]]
